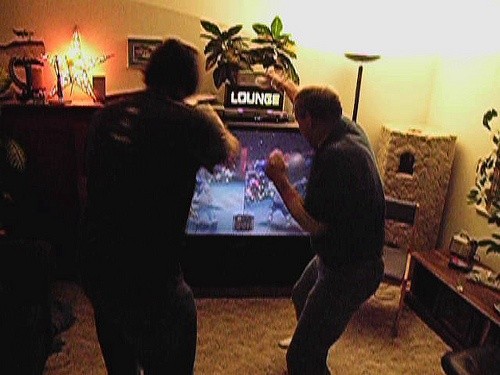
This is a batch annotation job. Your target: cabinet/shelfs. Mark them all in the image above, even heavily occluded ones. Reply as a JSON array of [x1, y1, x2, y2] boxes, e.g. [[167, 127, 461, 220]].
[[409, 243, 500, 351], [0, 98, 103, 245]]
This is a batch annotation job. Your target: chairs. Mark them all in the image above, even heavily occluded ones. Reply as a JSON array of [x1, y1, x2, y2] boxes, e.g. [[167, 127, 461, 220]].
[[366, 196, 420, 333]]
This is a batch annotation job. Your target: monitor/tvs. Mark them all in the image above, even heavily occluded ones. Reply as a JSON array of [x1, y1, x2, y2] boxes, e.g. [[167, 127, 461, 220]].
[[184, 120, 319, 248]]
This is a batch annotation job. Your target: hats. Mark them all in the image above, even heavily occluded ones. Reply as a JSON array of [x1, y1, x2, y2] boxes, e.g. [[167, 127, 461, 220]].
[[142, 37, 198, 98]]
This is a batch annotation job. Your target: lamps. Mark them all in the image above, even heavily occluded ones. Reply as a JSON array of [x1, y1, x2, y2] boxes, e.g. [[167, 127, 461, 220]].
[[344, 52, 379, 122]]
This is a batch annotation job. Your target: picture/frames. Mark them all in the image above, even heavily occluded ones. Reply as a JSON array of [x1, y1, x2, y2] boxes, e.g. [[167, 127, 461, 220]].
[[127, 37, 164, 72]]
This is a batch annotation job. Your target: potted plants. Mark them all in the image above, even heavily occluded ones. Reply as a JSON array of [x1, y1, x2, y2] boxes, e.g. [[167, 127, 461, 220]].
[[201, 16, 299, 90]]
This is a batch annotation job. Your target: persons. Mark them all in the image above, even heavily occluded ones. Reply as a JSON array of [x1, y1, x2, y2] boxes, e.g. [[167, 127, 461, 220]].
[[80, 38, 241, 375], [262, 75, 387, 375]]
